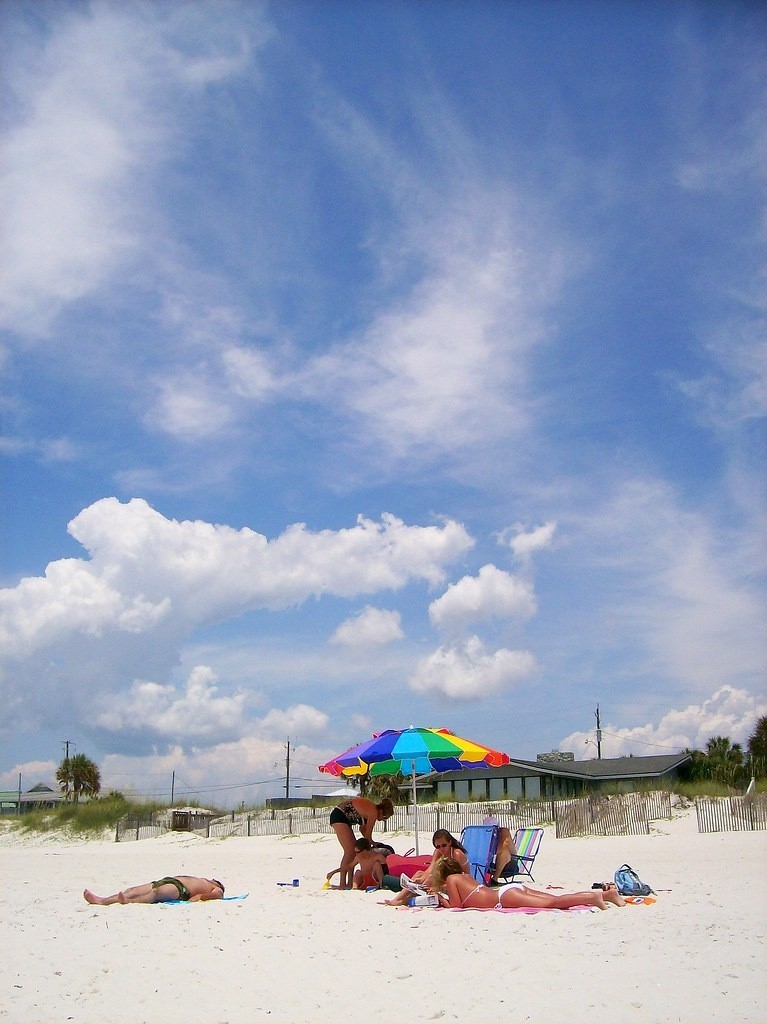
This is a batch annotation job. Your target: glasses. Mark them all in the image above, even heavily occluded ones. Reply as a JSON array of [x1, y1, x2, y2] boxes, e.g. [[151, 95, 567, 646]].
[[435, 840, 449, 849], [382, 808, 388, 820], [356, 849, 364, 855], [215, 881, 221, 888]]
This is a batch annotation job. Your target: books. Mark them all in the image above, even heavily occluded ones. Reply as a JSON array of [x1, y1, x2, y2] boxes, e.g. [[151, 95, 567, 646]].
[[400, 873, 440, 908]]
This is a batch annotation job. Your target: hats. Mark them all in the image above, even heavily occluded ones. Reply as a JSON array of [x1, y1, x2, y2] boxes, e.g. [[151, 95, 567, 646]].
[[483, 817, 498, 827]]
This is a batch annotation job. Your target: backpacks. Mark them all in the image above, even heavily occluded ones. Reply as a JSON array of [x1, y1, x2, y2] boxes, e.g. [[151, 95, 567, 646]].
[[614, 864, 658, 897]]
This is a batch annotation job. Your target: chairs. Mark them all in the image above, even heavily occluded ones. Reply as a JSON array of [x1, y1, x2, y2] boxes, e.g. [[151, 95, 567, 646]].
[[490, 828, 544, 883], [458, 825, 501, 886]]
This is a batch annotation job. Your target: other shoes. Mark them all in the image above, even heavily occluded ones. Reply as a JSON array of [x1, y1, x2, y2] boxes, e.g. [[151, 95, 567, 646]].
[[491, 878, 498, 886]]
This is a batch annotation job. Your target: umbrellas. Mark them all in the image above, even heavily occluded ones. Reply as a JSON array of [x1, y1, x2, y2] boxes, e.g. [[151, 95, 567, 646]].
[[318, 725, 510, 857]]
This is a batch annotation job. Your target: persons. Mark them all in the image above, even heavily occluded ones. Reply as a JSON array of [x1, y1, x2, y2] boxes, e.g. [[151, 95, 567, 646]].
[[428, 857, 626, 910], [84, 876, 224, 905], [330, 798, 394, 890], [483, 818, 518, 885], [385, 829, 470, 906], [327, 838, 386, 890]]
